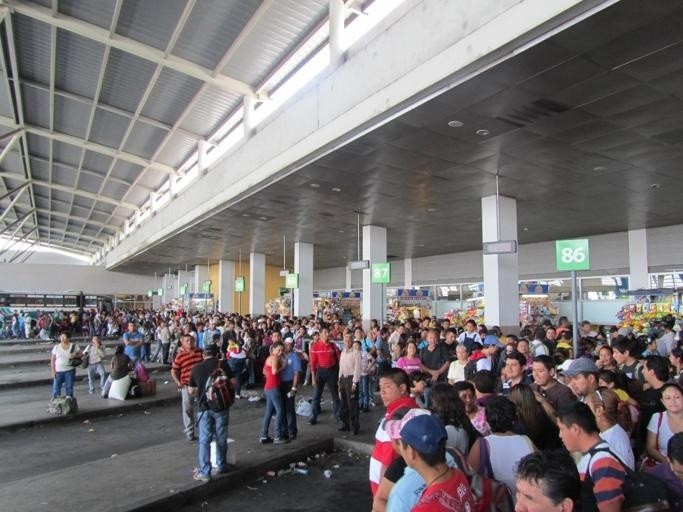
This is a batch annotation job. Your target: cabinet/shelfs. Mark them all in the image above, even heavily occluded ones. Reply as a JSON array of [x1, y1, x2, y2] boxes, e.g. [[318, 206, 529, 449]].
[[158, 298, 215, 312], [520, 295, 557, 325], [265, 292, 293, 321], [385, 295, 430, 319], [615, 289, 682, 327], [310, 297, 361, 323], [465, 295, 484, 324]]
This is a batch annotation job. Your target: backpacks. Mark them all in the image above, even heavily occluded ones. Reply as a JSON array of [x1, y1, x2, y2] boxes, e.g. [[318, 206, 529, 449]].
[[464, 357, 491, 379], [200, 359, 235, 413]]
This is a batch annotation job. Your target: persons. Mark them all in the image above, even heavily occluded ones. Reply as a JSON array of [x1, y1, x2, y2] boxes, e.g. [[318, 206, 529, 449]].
[[368, 317, 683, 512], [81, 335, 133, 398], [277, 338, 302, 442], [0, 309, 79, 342], [83, 303, 369, 386], [50, 331, 83, 397], [306, 327, 343, 426], [336, 335, 363, 433], [169, 334, 203, 440], [259, 341, 288, 444], [186, 343, 238, 484]]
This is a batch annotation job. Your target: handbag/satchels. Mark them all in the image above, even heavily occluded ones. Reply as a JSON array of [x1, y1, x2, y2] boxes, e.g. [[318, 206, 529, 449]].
[[49, 396, 78, 416], [367, 347, 377, 358], [637, 450, 659, 472], [449, 438, 491, 512], [67, 357, 82, 367], [585, 449, 682, 512], [80, 353, 89, 368], [491, 481, 515, 512]]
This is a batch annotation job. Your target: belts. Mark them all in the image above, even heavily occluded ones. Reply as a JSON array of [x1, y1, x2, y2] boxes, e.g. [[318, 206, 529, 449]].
[[340, 374, 354, 379]]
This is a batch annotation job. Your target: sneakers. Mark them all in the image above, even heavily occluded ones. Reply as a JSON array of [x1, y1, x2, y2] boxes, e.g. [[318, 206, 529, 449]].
[[192, 472, 211, 482], [308, 415, 317, 425]]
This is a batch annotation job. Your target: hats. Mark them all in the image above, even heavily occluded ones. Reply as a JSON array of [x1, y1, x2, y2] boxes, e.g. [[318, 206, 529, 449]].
[[399, 415, 448, 454], [484, 335, 504, 348], [283, 336, 294, 344], [560, 356, 600, 375], [385, 409, 431, 439], [557, 359, 572, 369]]
